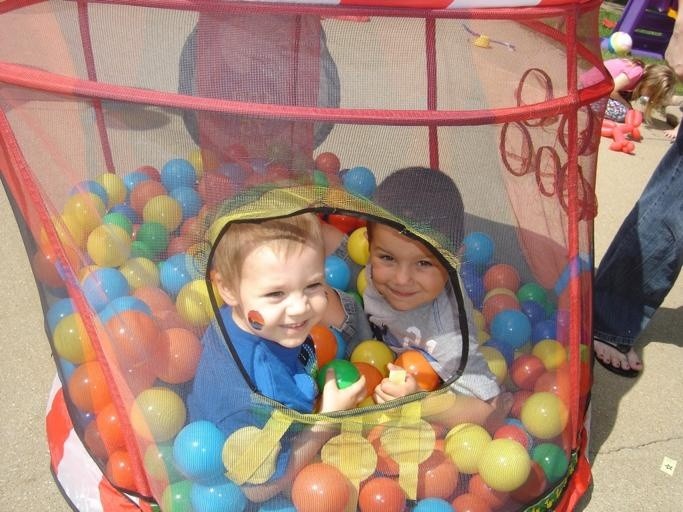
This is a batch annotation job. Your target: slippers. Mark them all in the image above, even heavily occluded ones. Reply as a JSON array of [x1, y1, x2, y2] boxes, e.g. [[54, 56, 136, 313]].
[[593, 337, 639, 377]]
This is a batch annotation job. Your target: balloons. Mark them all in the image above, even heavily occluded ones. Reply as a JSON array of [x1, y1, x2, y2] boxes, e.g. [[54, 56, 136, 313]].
[[592, 110, 645, 156], [609, 32, 633, 59]]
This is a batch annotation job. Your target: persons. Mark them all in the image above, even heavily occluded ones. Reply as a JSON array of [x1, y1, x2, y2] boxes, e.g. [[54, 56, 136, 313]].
[[571, 52, 683, 123], [185, 182, 367, 512], [588, 115, 683, 382], [320, 168, 513, 439]]
